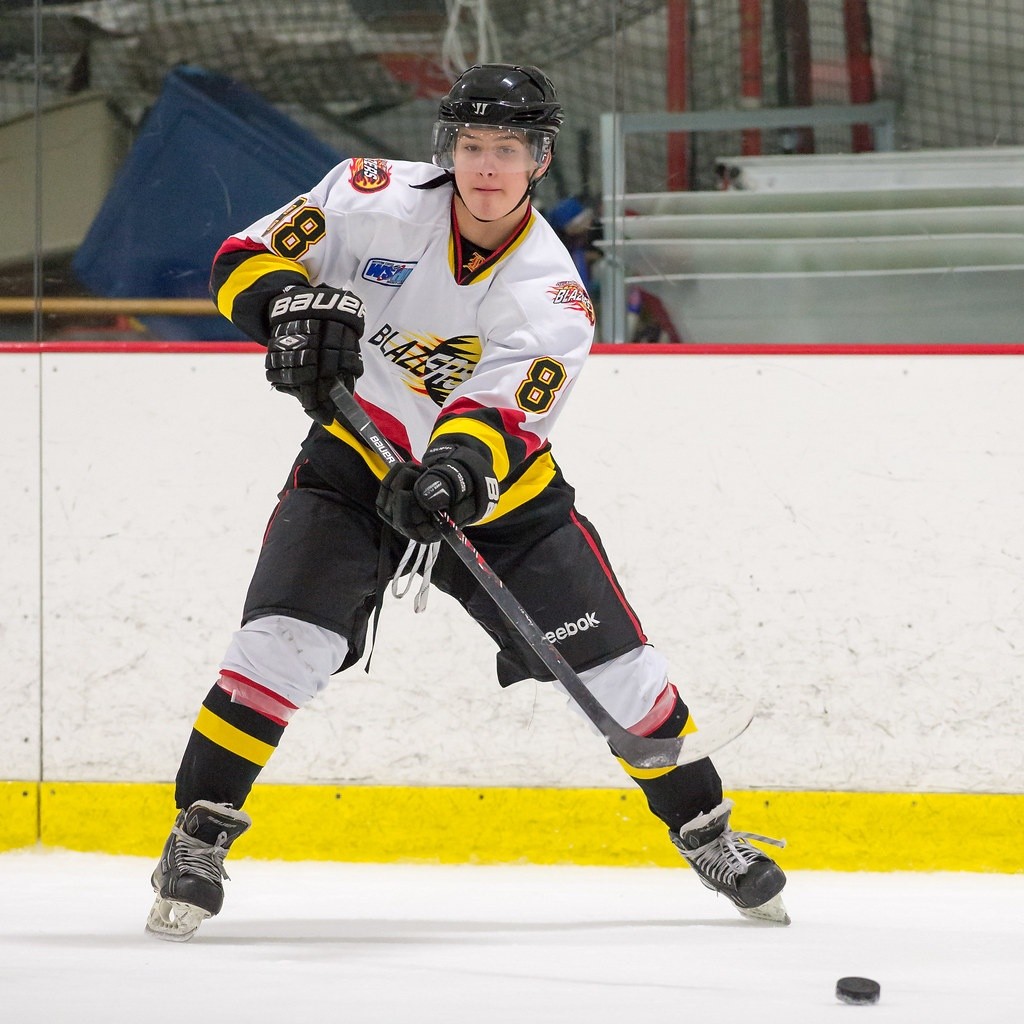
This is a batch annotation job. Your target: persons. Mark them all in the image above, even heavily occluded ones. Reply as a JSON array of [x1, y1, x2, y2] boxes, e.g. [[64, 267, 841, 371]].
[[142, 61, 797, 942]]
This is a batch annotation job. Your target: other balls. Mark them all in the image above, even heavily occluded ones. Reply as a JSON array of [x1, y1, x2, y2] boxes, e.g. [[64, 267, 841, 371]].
[[831, 970, 885, 1004]]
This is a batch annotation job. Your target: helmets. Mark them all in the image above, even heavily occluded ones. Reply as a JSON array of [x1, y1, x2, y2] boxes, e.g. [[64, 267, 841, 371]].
[[433, 63, 562, 173]]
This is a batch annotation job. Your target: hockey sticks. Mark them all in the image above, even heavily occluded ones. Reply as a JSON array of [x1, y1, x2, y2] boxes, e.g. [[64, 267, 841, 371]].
[[325, 374, 757, 772]]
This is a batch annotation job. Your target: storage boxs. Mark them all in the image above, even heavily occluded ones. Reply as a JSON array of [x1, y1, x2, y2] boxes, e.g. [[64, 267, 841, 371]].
[[72, 64, 343, 341], [1, 94, 136, 270]]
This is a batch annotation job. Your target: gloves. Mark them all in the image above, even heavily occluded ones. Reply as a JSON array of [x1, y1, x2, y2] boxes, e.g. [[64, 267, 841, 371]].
[[265, 282, 367, 426], [376, 443, 500, 544]]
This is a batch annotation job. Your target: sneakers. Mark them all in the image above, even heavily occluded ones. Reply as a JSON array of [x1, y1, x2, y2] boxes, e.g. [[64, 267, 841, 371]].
[[144, 800, 251, 942], [668, 797, 791, 926]]
